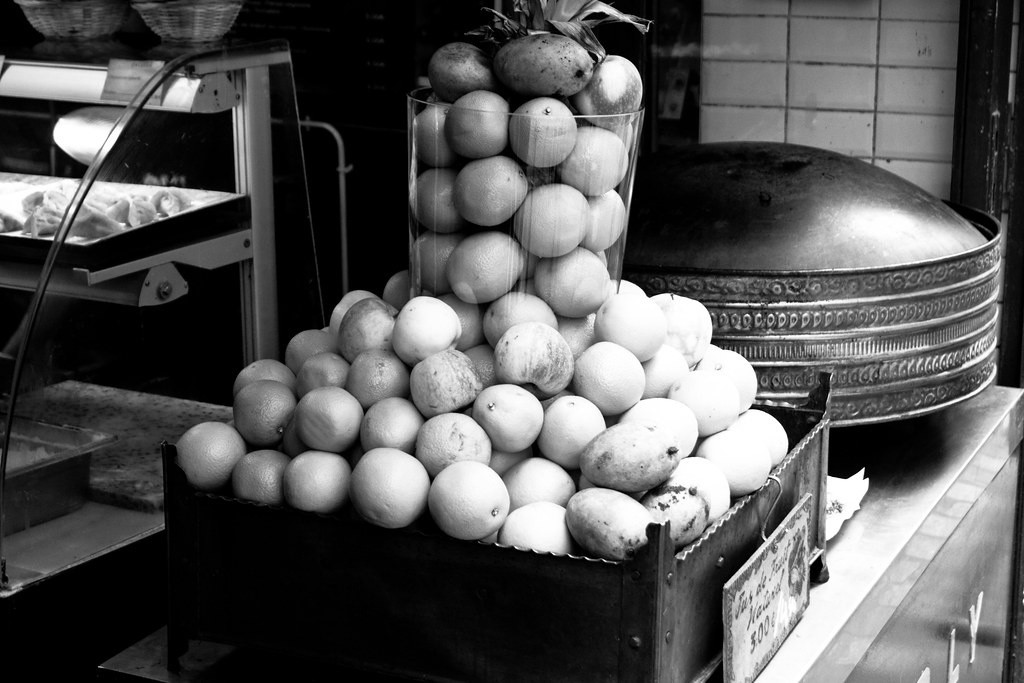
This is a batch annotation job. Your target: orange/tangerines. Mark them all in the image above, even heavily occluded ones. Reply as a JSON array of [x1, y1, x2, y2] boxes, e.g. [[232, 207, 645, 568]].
[[174, 33, 789, 561]]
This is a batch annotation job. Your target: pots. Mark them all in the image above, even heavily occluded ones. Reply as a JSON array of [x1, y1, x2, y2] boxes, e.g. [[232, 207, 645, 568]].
[[622, 140, 1004, 427]]
